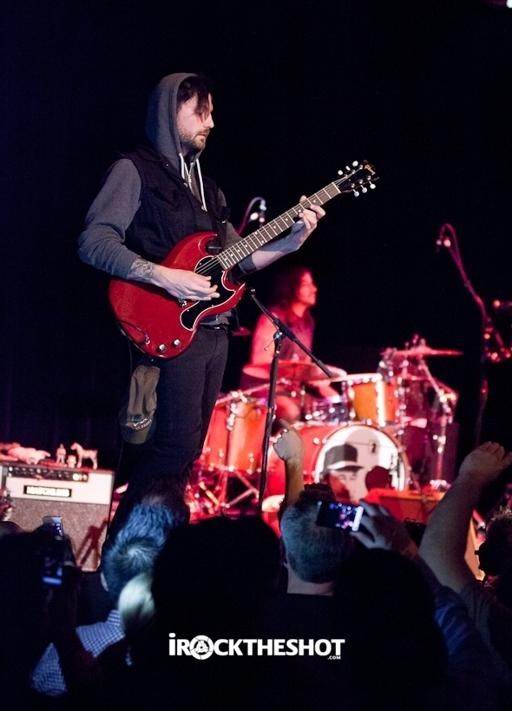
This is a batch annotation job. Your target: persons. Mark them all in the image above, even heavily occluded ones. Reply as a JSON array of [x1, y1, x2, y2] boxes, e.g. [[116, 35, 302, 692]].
[[74, 70, 328, 567], [2, 417, 511, 711], [238, 265, 342, 426]]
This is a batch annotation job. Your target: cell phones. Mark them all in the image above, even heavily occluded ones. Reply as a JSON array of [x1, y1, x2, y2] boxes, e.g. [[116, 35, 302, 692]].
[[317, 500, 364, 532], [41, 517, 65, 584]]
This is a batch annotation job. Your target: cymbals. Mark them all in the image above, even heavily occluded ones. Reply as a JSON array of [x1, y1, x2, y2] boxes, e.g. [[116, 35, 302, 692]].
[[243, 361, 345, 384], [380, 349, 463, 359]]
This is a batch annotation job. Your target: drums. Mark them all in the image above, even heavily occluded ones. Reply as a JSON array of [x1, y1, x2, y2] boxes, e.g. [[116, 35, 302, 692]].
[[261, 418, 412, 512], [339, 372, 407, 430], [305, 395, 350, 422], [191, 389, 278, 481]]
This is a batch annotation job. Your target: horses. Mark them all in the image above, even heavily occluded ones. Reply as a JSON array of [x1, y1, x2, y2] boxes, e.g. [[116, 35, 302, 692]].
[[71, 442, 98, 470]]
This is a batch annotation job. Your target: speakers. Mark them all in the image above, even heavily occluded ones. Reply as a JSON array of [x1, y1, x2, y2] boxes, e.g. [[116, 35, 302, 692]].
[[0, 460, 115, 571]]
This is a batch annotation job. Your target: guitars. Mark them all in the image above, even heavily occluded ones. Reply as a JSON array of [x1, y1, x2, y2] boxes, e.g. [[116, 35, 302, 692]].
[[109, 161, 379, 359]]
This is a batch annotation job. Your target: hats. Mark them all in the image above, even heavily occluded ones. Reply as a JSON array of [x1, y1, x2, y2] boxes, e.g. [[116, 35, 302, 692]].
[[118, 365, 161, 444]]
